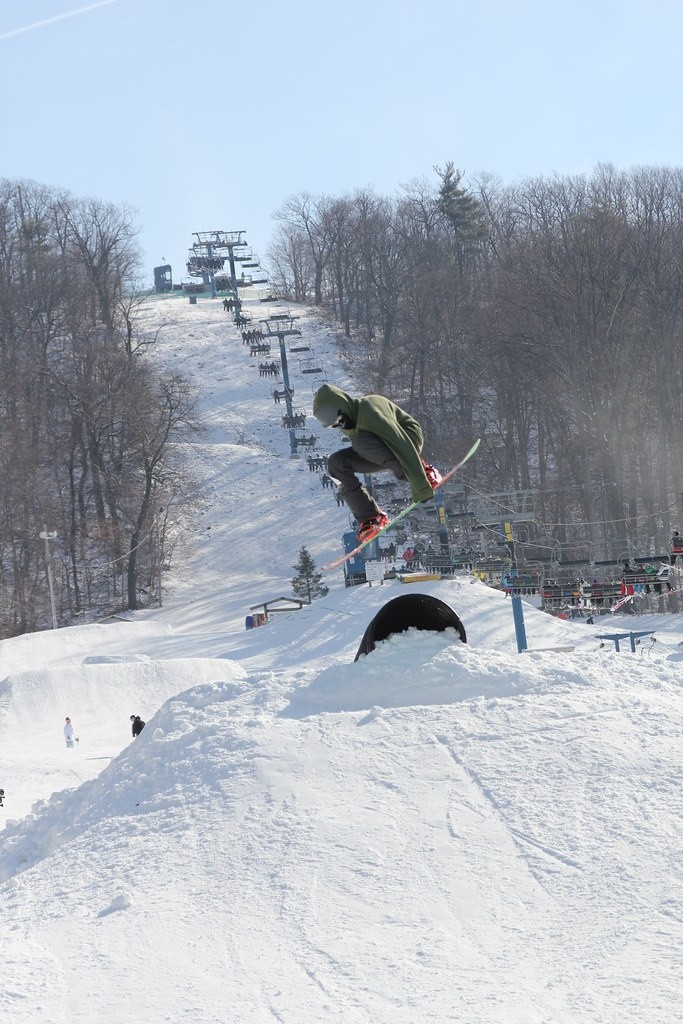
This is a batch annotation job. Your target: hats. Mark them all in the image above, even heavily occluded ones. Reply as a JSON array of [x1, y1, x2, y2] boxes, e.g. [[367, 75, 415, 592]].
[[315, 408, 338, 429]]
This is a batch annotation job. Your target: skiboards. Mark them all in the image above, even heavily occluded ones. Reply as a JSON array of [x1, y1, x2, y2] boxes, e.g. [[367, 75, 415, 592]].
[[610, 587, 683, 612], [660, 561, 683, 573]]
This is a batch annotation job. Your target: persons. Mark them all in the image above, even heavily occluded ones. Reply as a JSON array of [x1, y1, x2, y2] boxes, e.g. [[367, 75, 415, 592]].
[[379, 543, 482, 575], [620, 562, 675, 595], [243, 328, 269, 354], [545, 580, 561, 604], [273, 388, 307, 428], [671, 531, 683, 563], [310, 455, 357, 530], [566, 578, 623, 607], [190, 256, 225, 270], [301, 434, 315, 450], [64, 717, 74, 747], [505, 572, 538, 594], [223, 297, 245, 327], [313, 384, 441, 541], [130, 715, 145, 737], [217, 277, 232, 292], [587, 617, 593, 624], [478, 554, 511, 580], [261, 362, 279, 376], [0, 789, 5, 806]]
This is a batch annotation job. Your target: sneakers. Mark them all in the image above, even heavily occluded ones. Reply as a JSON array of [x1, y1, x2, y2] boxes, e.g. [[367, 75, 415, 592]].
[[424, 465, 442, 488], [357, 512, 388, 541]]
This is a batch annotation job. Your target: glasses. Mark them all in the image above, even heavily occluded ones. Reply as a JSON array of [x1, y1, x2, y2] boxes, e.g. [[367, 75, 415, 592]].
[[327, 416, 346, 429]]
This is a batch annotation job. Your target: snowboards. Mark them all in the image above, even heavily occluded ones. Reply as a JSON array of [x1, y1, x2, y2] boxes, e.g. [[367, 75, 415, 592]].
[[567, 606, 600, 610], [321, 438, 480, 571]]
